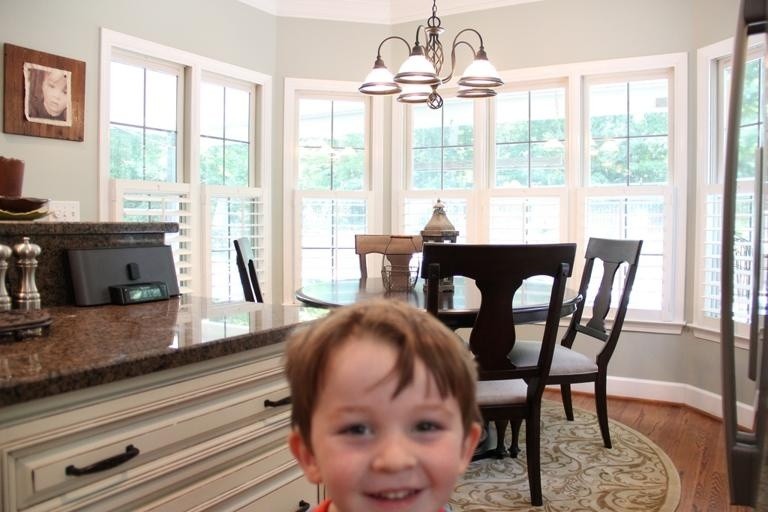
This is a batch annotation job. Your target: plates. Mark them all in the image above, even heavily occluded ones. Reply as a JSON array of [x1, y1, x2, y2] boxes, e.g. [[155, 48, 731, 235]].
[[0, 197, 48, 211], [0, 211, 57, 221]]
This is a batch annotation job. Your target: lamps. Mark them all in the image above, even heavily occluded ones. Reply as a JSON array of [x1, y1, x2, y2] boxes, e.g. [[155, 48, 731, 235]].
[[358, 1, 504, 109]]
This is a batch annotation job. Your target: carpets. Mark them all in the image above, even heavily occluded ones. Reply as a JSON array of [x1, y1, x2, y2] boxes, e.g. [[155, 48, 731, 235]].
[[447, 398, 682, 512]]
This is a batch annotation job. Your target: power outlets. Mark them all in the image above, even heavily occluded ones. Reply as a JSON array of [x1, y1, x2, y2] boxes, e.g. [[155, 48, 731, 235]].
[[49, 201, 81, 222]]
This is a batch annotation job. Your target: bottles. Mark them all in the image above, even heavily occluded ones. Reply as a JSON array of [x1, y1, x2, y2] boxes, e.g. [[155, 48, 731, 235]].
[[13, 236, 41, 309], [0, 245, 12, 311]]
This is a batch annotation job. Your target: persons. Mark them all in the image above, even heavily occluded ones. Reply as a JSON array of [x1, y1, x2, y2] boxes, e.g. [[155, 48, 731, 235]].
[[29, 71, 68, 122], [283, 298, 484, 512]]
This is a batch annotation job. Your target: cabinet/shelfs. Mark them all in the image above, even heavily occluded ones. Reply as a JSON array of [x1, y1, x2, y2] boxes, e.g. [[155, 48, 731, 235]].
[[0, 341, 326, 512]]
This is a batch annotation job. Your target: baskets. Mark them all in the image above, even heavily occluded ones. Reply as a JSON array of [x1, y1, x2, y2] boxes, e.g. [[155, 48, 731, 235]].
[[381, 236, 420, 293]]
